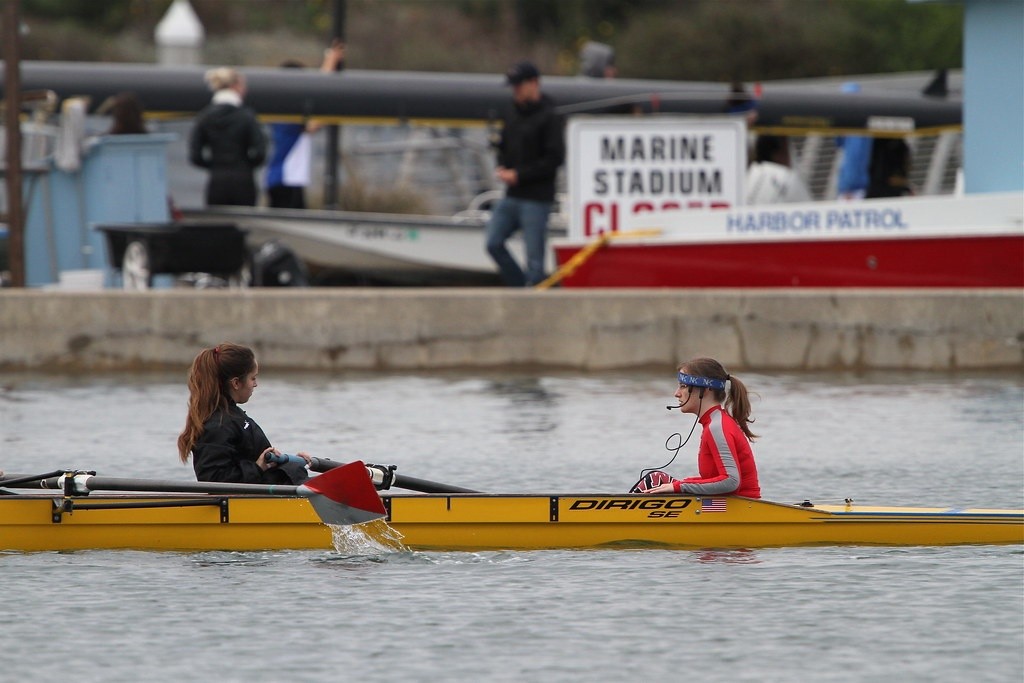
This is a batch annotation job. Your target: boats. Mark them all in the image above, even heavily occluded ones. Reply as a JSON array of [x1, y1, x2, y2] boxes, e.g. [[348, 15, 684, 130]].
[[0, 492, 1022, 549], [168, 191, 568, 285]]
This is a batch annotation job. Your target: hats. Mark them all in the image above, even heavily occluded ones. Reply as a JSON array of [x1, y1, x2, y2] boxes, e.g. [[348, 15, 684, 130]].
[[505, 63, 539, 87]]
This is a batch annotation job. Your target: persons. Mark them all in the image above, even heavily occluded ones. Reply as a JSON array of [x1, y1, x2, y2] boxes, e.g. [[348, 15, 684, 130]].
[[745, 111, 810, 203], [177, 343, 311, 483], [576, 43, 619, 78], [188, 69, 270, 285], [95, 94, 151, 134], [632, 358, 762, 499], [921, 67, 951, 99], [242, 42, 346, 287], [867, 135, 912, 197], [487, 63, 563, 285], [832, 82, 870, 200]]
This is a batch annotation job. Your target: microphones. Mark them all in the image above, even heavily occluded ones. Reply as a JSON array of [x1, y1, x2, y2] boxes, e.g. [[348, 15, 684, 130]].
[[667, 386, 692, 410]]
